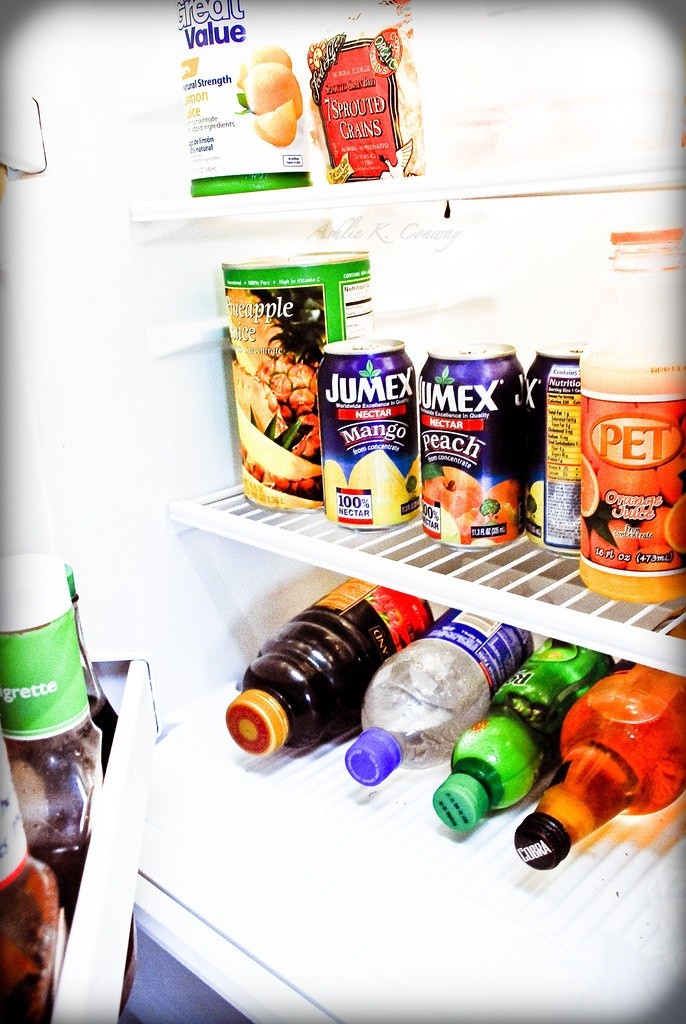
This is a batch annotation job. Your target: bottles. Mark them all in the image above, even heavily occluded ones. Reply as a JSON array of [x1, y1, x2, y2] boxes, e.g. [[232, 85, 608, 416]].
[[221, 575, 436, 762], [1, 731, 66, 1024], [1, 553, 136, 1017], [431, 636, 634, 836], [53, 565, 120, 777], [514, 603, 686, 872], [341, 606, 534, 792], [571, 213, 685, 607]]
